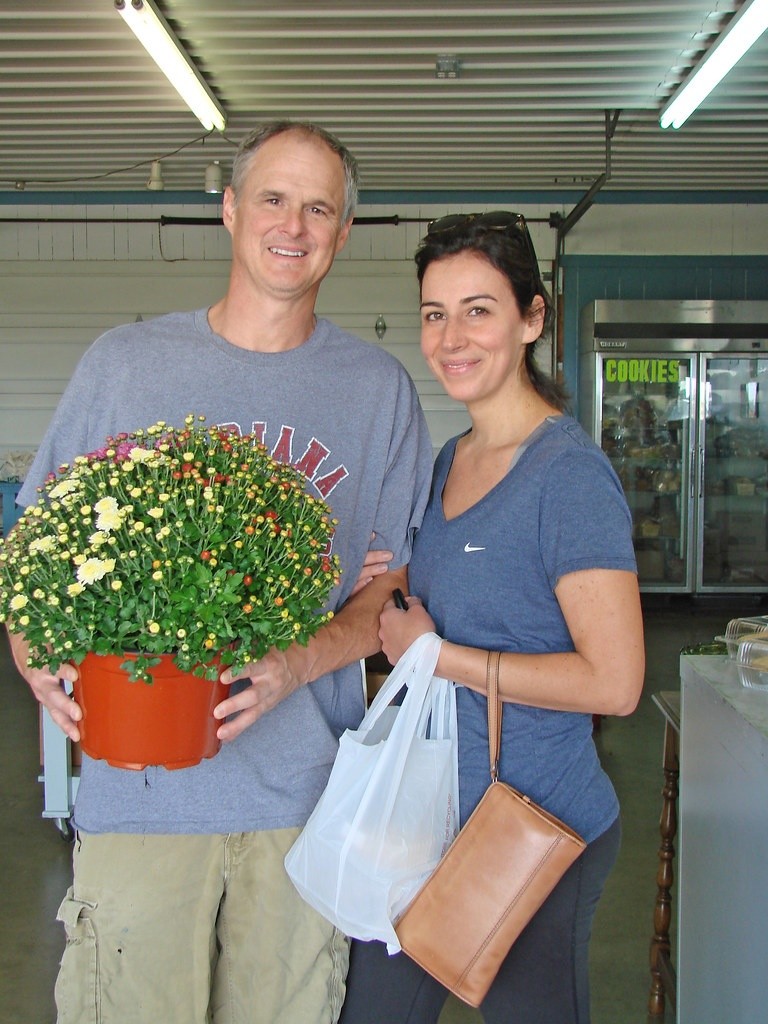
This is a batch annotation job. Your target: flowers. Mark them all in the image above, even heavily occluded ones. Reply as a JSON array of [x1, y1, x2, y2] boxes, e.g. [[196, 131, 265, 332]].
[[0, 416, 339, 679]]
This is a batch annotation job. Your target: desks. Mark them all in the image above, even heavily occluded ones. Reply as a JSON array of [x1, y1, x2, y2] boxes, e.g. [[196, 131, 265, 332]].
[[652, 651, 768, 1024]]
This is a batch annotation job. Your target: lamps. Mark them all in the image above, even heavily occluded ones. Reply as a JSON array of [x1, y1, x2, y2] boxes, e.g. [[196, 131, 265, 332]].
[[658, 0, 768, 129], [148, 162, 164, 190], [114, 0, 229, 131], [204, 161, 224, 193]]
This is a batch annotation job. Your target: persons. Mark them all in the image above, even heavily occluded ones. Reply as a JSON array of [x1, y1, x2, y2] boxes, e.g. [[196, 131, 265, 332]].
[[338, 211, 645, 1024], [0, 119, 434, 1024]]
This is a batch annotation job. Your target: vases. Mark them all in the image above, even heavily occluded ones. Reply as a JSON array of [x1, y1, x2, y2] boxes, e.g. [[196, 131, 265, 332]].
[[68, 639, 240, 772]]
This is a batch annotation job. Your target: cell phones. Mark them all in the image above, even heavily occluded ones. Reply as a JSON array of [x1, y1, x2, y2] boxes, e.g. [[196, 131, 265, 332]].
[[392, 587, 408, 613]]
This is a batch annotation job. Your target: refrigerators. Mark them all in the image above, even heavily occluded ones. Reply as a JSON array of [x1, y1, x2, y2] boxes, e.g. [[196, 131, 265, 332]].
[[580, 300, 768, 613]]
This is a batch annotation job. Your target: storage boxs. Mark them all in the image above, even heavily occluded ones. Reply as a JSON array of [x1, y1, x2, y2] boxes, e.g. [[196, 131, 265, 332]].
[[725, 640, 768, 692], [714, 615, 768, 660]]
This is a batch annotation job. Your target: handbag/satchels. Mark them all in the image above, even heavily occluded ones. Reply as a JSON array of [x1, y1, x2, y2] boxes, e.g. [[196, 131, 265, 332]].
[[284, 633, 460, 956], [393, 651, 587, 1009]]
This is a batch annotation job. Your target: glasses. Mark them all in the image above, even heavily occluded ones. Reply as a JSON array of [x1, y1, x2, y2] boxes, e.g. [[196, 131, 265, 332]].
[[428, 210, 542, 299]]
